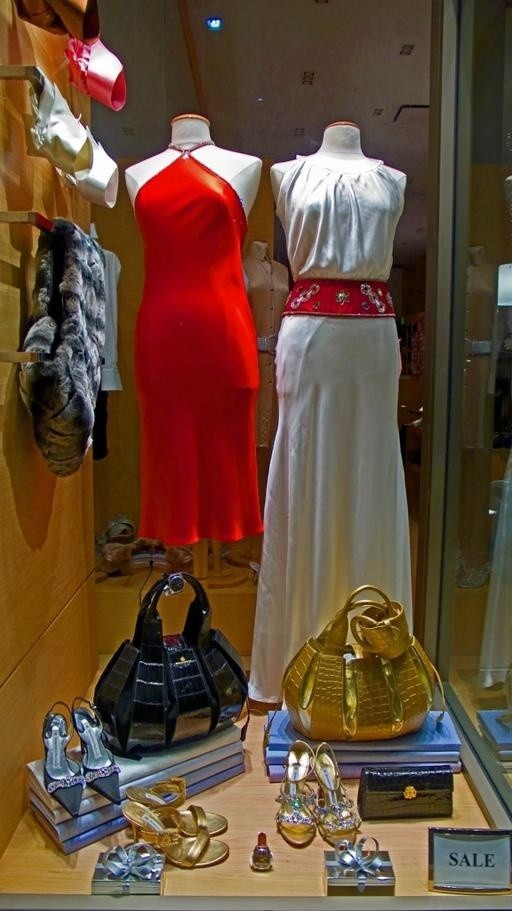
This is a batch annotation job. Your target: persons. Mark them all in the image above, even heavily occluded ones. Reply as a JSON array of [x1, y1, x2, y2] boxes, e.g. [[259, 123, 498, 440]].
[[247, 119, 414, 703], [239, 239, 289, 447], [124, 112, 265, 547]]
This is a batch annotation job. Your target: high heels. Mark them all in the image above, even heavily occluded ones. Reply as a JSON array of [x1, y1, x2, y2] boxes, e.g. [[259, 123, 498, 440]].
[[121, 800, 230, 870], [41, 699, 85, 818], [309, 740, 363, 852], [273, 738, 320, 848], [71, 696, 123, 806], [125, 775, 229, 837]]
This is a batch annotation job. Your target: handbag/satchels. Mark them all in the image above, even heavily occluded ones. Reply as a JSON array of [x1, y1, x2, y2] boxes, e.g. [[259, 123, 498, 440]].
[[93, 570, 250, 760], [357, 765, 454, 821], [283, 584, 445, 743]]
